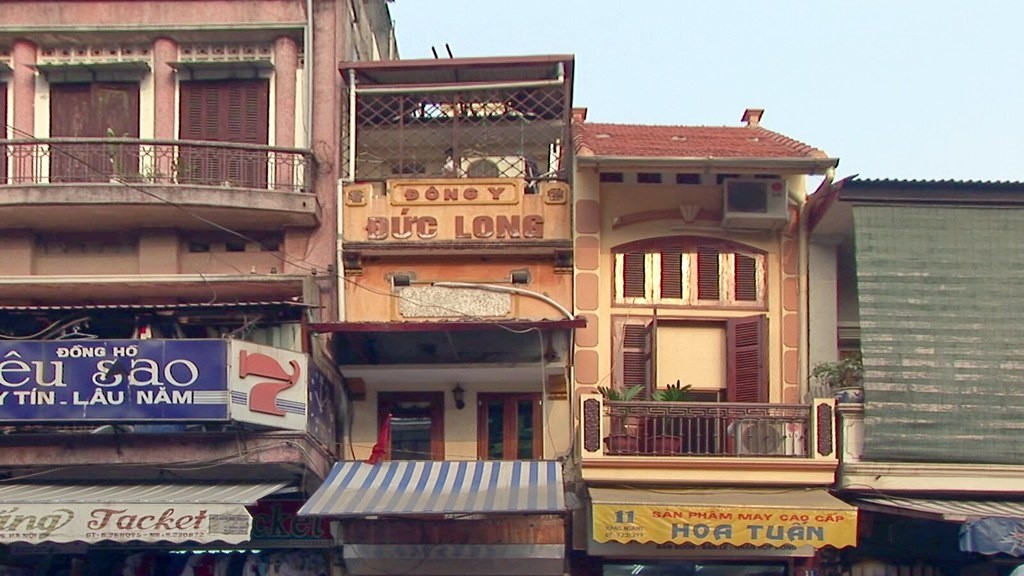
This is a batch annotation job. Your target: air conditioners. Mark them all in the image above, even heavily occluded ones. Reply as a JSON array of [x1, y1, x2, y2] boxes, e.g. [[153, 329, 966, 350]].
[[723, 176, 788, 231]]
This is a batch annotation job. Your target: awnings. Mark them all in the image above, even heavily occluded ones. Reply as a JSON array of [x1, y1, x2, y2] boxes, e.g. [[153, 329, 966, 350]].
[[588, 488, 857, 549], [0, 479, 294, 546], [860, 499, 1024, 558], [297, 460, 565, 514]]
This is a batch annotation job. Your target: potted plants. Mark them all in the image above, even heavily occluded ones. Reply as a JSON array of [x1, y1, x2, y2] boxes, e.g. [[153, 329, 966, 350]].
[[648, 378, 696, 452], [601, 381, 643, 452], [807, 354, 864, 404]]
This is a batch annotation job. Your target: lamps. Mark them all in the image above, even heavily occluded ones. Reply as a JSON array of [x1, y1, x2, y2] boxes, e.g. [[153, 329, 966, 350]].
[[511, 272, 530, 283], [451, 383, 465, 410], [394, 276, 411, 286]]
